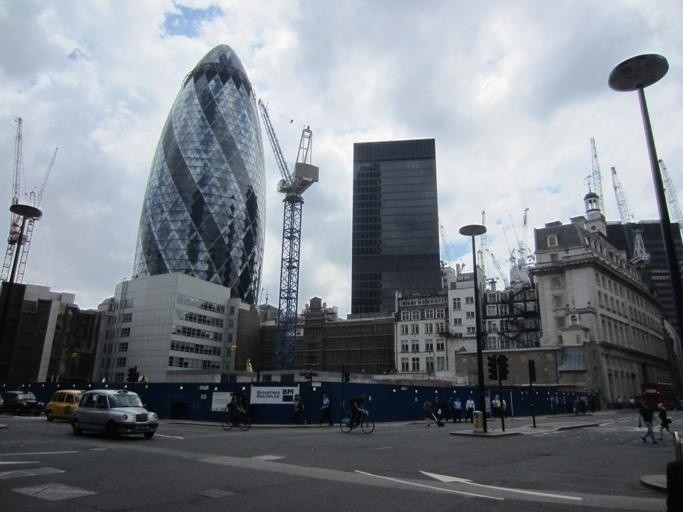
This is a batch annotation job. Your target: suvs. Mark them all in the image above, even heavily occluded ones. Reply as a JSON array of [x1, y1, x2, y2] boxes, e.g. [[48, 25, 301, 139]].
[[2, 391, 45, 416]]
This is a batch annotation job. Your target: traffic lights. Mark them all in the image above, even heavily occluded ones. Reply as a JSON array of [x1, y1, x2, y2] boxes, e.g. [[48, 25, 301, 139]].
[[527, 358, 537, 382], [487, 354, 509, 380]]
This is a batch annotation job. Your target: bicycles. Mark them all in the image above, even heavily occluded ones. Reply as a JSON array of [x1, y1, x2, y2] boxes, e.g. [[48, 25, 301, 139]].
[[220, 409, 252, 431], [339, 410, 375, 434]]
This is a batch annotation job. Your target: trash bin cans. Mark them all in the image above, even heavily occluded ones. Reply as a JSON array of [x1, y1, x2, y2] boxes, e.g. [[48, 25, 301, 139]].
[[472, 411, 484, 432], [665, 430, 683, 512]]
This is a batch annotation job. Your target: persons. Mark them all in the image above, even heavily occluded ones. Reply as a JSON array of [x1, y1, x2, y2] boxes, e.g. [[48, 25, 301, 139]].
[[292, 394, 304, 423], [454, 397, 461, 422], [228, 391, 246, 427], [465, 396, 474, 422], [492, 394, 506, 418], [318, 395, 334, 427], [424, 400, 431, 424], [612, 395, 641, 408], [638, 398, 676, 443], [349, 395, 367, 427]]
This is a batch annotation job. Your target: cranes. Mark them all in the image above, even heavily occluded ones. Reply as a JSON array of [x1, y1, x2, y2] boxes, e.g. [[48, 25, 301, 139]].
[[257, 98, 318, 370], [0, 118, 58, 284], [583, 137, 683, 264], [440, 207, 535, 291]]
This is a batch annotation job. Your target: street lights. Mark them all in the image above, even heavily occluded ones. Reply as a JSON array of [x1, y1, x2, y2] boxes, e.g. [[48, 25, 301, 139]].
[[458, 225, 487, 432], [607, 53, 680, 338]]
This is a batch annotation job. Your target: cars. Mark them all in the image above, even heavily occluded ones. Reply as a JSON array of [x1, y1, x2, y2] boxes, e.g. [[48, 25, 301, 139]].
[[44, 389, 86, 421], [69, 389, 159, 439]]
